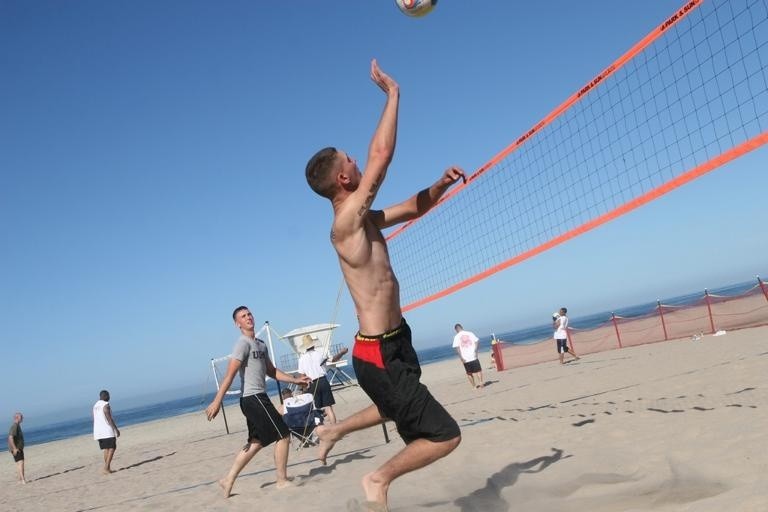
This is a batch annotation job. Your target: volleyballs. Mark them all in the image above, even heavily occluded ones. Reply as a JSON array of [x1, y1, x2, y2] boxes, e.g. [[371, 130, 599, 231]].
[[394, 0, 438, 16]]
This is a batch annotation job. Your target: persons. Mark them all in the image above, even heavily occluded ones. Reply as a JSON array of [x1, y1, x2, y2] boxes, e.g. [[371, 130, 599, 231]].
[[551, 307, 581, 365], [204, 305, 314, 499], [92, 390, 120, 474], [8, 413, 29, 485], [276, 387, 295, 415], [451, 322, 487, 390], [297, 334, 349, 425], [305, 58, 469, 509]]
